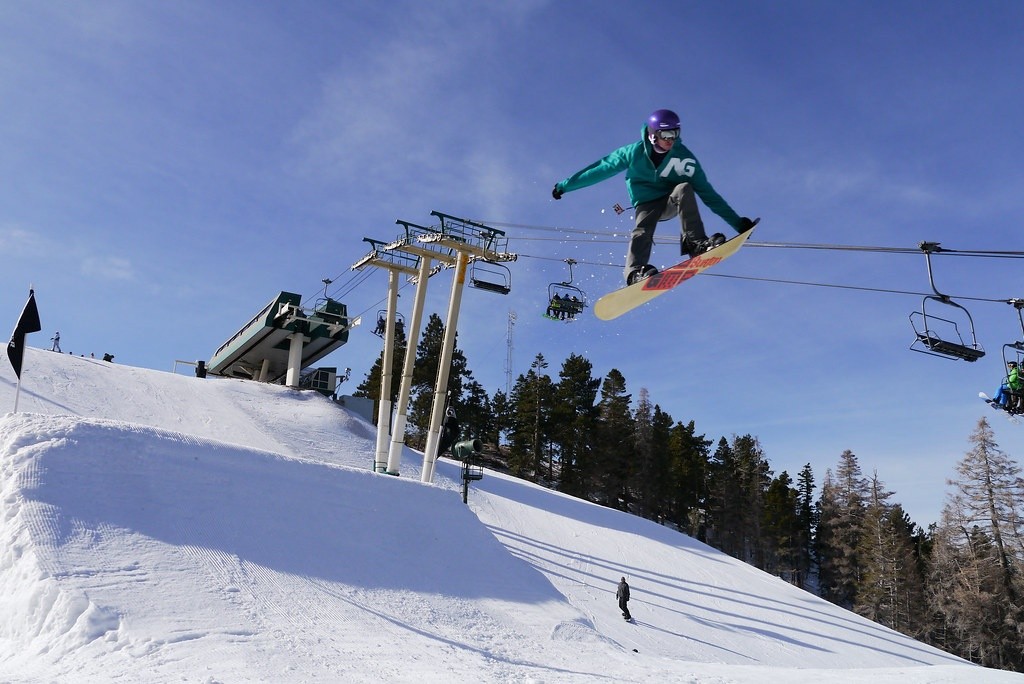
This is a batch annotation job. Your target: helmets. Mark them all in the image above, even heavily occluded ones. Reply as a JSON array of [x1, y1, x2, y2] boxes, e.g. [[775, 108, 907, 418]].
[[648, 109, 682, 140], [554, 293, 579, 300]]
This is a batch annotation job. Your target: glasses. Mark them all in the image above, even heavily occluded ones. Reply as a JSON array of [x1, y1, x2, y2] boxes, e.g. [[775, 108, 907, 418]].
[[656, 129, 680, 139]]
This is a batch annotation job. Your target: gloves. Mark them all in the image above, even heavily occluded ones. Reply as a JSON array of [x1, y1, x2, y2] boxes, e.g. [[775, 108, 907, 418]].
[[552, 183, 564, 200], [737, 217, 752, 239]]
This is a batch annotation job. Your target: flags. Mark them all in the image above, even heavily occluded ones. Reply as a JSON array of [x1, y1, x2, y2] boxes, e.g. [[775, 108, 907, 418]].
[[7, 289, 41, 380]]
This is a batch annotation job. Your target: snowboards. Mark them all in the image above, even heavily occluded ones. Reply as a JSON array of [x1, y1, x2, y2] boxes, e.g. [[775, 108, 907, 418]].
[[46, 348, 63, 354], [594, 217, 761, 322], [625, 618, 631, 622]]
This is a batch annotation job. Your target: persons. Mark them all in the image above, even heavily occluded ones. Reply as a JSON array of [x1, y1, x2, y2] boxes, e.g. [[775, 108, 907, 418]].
[[616, 576, 632, 620], [551, 109, 754, 287], [373, 315, 403, 338], [546, 293, 580, 321], [991, 361, 1024, 415], [50, 330, 61, 352]]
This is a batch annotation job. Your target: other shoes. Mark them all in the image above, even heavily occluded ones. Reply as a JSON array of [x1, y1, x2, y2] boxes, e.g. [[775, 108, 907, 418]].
[[544, 314, 578, 323]]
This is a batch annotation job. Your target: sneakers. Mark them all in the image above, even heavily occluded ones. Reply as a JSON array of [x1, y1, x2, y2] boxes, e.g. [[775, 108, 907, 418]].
[[630, 265, 659, 284], [690, 232, 726, 260]]
